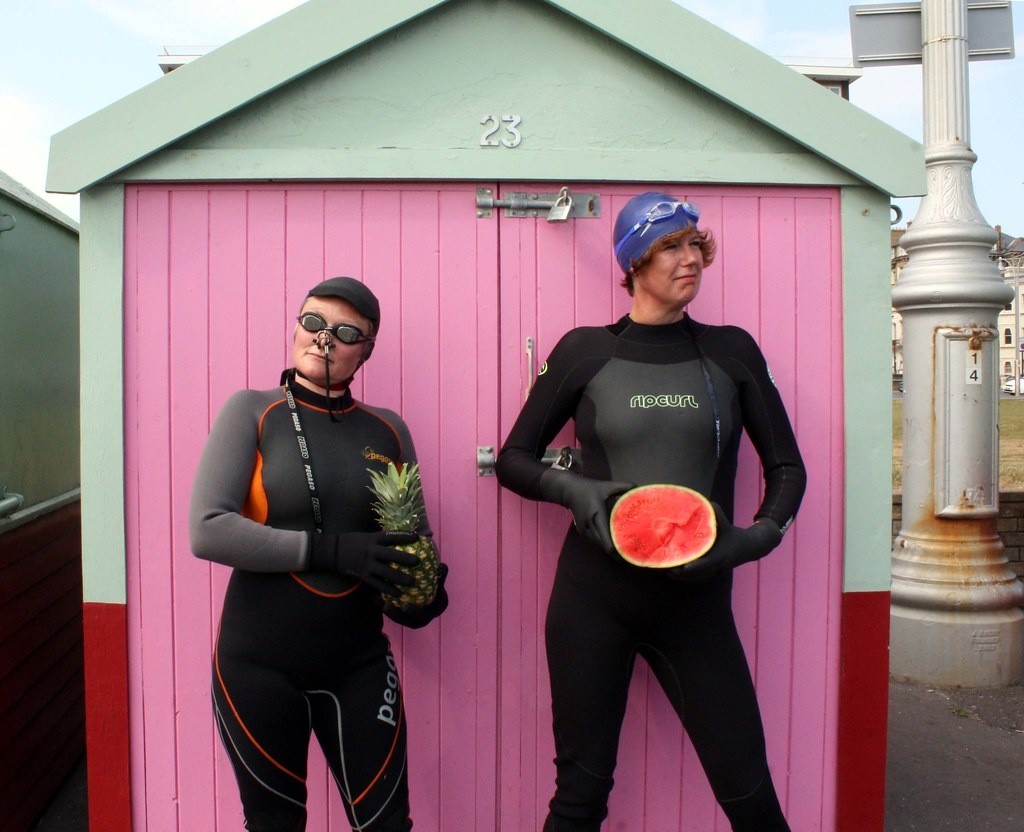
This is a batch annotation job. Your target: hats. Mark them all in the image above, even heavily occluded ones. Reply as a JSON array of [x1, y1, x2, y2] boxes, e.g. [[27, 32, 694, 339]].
[[309, 276, 381, 323], [613, 192, 698, 273]]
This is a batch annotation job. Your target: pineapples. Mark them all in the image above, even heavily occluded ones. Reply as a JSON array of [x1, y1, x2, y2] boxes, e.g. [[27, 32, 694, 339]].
[[364, 462, 440, 609]]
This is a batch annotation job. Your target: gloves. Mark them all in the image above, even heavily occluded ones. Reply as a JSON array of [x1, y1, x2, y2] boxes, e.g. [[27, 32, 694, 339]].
[[382, 563, 449, 630], [331, 528, 422, 599], [538, 467, 637, 555], [667, 499, 784, 586]]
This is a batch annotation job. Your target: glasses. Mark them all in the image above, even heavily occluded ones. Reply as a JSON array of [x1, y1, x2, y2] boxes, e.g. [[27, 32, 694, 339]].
[[296, 313, 373, 345], [646, 200, 701, 222]]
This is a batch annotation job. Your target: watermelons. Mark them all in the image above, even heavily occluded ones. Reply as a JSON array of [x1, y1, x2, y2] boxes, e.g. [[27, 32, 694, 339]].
[[609, 483, 718, 568]]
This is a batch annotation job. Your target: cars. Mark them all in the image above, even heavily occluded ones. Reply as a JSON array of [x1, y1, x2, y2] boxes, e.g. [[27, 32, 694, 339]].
[[898, 382, 903, 392], [1003, 375, 1024, 395]]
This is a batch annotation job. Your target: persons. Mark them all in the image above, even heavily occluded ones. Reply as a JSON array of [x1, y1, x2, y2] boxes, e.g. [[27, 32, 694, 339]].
[[189, 276, 449, 832], [495, 191, 807, 832]]
